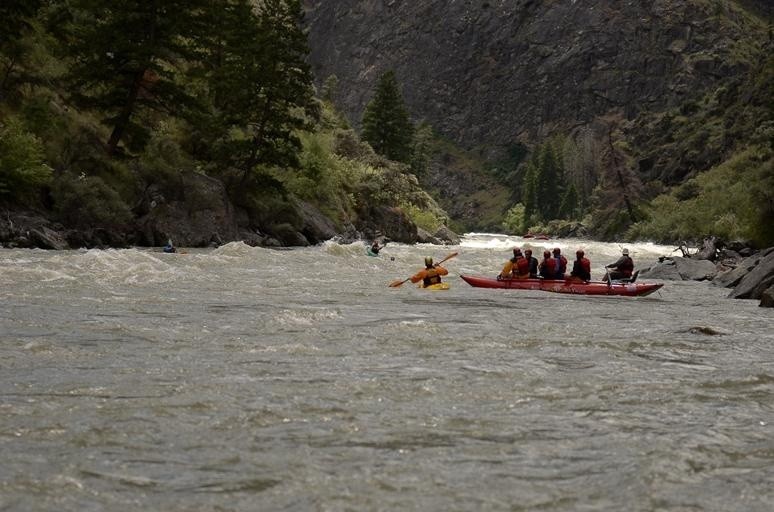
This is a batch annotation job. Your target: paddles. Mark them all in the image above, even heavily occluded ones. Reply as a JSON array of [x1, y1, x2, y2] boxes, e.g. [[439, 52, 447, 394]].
[[389, 253, 458, 288]]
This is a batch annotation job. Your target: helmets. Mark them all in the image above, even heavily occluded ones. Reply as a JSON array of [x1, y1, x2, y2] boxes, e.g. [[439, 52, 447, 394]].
[[425, 256, 433, 266]]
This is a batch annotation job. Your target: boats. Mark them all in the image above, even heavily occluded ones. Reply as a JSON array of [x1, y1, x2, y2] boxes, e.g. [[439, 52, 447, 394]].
[[366, 246, 382, 259], [459, 272, 664, 297], [534, 235, 548, 240], [417, 280, 449, 291], [523, 233, 533, 238]]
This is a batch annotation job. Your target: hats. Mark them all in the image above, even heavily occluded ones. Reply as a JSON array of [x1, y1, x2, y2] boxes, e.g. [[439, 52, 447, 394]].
[[621, 248, 629, 254], [552, 248, 561, 253], [576, 250, 584, 256], [544, 251, 551, 257]]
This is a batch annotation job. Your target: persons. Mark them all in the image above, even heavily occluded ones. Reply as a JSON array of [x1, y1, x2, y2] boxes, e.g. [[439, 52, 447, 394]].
[[570, 249, 592, 279], [601, 248, 633, 282], [523, 249, 538, 276], [552, 248, 568, 278], [500, 247, 531, 279], [372, 240, 386, 255], [408, 257, 448, 286], [537, 252, 554, 278]]
